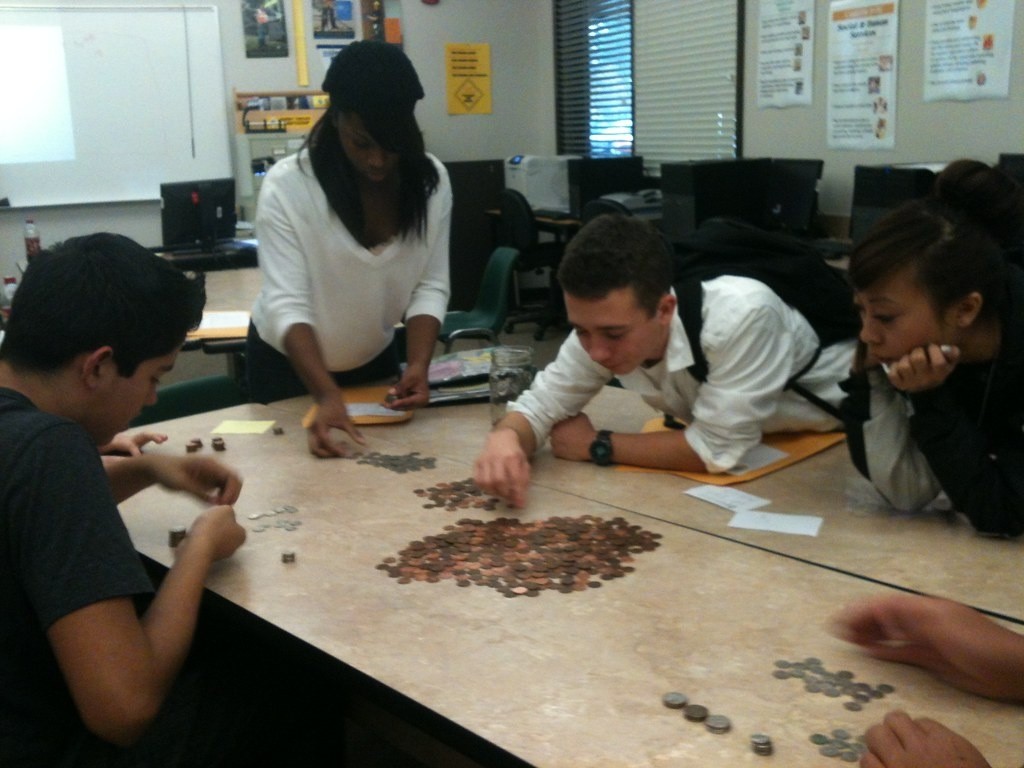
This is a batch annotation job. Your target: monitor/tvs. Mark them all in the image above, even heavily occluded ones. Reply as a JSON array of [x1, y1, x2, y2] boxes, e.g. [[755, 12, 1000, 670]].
[[847, 166, 932, 243], [761, 159, 827, 239], [159, 178, 236, 255]]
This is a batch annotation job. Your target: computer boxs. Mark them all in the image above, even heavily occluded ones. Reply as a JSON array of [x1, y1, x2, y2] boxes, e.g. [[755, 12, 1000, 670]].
[[568, 157, 643, 220], [661, 157, 768, 239]]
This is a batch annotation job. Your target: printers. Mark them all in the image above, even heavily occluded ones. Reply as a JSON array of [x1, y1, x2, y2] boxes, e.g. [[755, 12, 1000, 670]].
[[504, 153, 582, 214]]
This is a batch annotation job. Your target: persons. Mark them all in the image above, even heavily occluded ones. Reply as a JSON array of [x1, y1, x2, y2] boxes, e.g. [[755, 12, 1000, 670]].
[[238, 39, 453, 458], [828, 592, 1024, 768], [0, 230, 247, 768], [837, 160, 1024, 539], [473, 215, 846, 508]]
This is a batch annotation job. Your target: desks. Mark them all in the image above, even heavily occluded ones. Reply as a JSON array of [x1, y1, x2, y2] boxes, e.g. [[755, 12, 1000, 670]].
[[157, 238, 264, 270], [485, 207, 583, 303], [180, 312, 407, 394]]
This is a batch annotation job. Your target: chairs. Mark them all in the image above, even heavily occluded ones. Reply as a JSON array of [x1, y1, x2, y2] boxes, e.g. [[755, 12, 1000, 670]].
[[438, 187, 864, 354], [438, 245, 519, 355], [580, 199, 631, 227], [128, 374, 253, 430], [101, 377, 1023, 768], [470, 188, 568, 341]]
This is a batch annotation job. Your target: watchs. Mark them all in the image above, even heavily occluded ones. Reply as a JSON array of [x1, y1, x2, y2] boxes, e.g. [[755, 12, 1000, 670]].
[[589, 430, 614, 466]]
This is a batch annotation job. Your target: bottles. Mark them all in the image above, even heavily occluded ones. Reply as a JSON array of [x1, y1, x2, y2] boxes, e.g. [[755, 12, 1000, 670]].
[[25, 220, 41, 264], [0, 277, 19, 325]]
